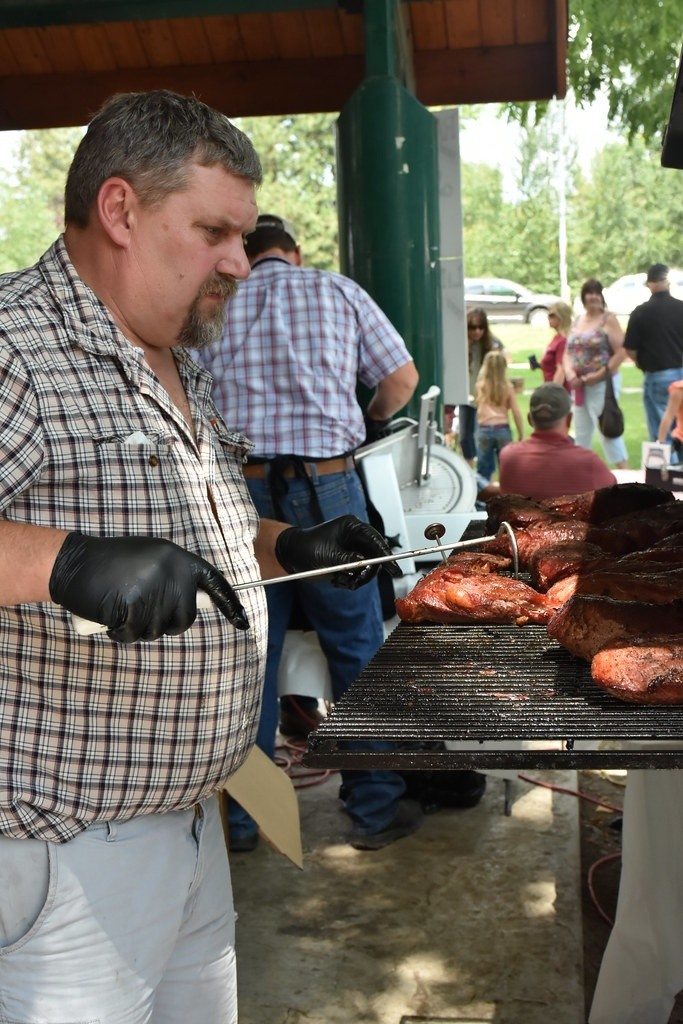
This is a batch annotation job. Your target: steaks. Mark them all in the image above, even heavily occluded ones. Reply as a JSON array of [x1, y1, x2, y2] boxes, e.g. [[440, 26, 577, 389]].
[[392, 482, 683, 704]]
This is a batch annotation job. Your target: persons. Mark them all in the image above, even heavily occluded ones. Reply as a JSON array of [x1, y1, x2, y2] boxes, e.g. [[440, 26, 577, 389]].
[[465, 263, 683, 505], [186, 215, 487, 851], [0, 89, 403, 1024]]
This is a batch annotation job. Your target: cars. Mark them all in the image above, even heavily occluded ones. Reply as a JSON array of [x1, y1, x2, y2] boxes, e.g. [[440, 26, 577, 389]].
[[574, 273, 683, 317], [463, 278, 571, 328]]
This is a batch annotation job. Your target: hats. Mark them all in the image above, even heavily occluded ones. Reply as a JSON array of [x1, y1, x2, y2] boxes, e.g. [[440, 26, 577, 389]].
[[648, 264, 668, 281], [531, 384, 570, 422], [554, 299, 572, 323], [247, 214, 297, 252]]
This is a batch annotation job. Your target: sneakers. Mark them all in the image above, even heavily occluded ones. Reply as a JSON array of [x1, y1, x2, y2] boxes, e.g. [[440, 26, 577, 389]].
[[349, 801, 426, 851]]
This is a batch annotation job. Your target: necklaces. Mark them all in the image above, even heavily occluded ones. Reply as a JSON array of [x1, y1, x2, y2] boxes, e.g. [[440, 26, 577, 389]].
[[251, 257, 291, 269]]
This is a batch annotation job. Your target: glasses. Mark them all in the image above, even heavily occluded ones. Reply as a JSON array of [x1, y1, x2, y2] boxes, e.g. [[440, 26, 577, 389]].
[[469, 322, 485, 329], [549, 313, 555, 318]]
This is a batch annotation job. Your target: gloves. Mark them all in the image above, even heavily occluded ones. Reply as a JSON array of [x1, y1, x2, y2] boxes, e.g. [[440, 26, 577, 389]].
[[47, 531, 250, 642], [274, 515, 404, 588]]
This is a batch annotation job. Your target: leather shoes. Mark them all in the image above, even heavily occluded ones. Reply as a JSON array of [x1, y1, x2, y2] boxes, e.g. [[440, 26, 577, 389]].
[[241, 455, 355, 479]]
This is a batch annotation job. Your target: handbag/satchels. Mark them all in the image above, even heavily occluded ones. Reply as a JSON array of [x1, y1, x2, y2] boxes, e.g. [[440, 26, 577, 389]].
[[599, 361, 624, 438]]
